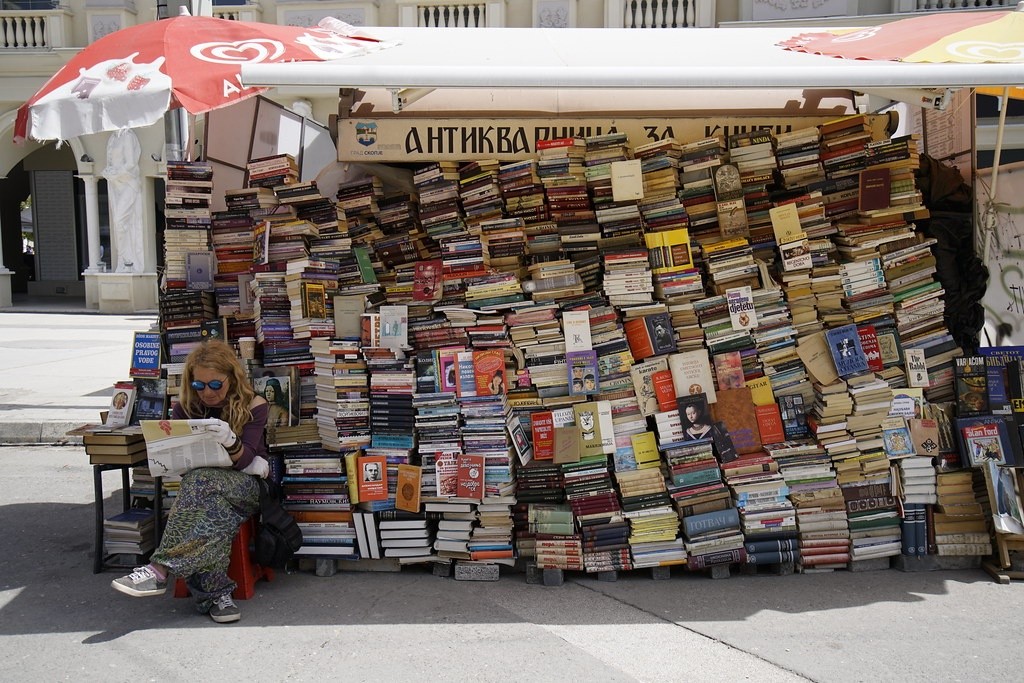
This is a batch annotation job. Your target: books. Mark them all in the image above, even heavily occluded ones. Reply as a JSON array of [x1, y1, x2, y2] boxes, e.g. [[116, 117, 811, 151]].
[[278, 451, 519, 568], [204, 152, 351, 277], [602, 253, 966, 472], [835, 467, 993, 561], [774, 108, 930, 239], [333, 161, 485, 288], [164, 158, 215, 287], [103, 271, 339, 430], [511, 428, 852, 570], [309, 260, 617, 455], [455, 133, 653, 306], [629, 128, 779, 303], [951, 352, 1024, 535]]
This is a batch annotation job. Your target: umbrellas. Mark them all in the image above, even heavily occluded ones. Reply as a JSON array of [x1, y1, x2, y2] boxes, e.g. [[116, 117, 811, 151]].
[[777, 11, 1024, 268], [12, 6, 391, 160]]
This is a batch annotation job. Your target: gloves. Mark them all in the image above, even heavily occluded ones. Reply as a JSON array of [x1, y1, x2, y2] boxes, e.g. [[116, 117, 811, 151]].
[[239, 454, 270, 478], [201, 416, 242, 455]]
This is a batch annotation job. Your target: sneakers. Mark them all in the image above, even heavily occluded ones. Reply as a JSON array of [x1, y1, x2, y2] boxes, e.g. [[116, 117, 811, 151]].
[[210, 595, 241, 622], [111, 566, 168, 597]]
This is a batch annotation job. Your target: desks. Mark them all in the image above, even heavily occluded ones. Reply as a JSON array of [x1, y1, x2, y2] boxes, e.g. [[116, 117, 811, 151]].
[[94, 458, 162, 573]]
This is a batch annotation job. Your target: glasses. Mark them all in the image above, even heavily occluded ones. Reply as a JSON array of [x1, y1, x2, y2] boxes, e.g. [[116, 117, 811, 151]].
[[191, 377, 230, 390]]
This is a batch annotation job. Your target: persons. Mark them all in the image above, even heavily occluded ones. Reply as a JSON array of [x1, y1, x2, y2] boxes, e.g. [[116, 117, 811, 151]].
[[488, 370, 504, 395], [100, 126, 146, 273], [364, 463, 380, 482], [654, 323, 672, 349], [682, 401, 712, 440], [108, 341, 270, 623]]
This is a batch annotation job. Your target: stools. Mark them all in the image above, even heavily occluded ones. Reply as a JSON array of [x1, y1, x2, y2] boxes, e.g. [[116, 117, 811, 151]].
[[175, 523, 274, 600]]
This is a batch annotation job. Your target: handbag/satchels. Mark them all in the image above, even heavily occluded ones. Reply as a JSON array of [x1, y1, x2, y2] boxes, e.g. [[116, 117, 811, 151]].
[[252, 494, 303, 569]]
